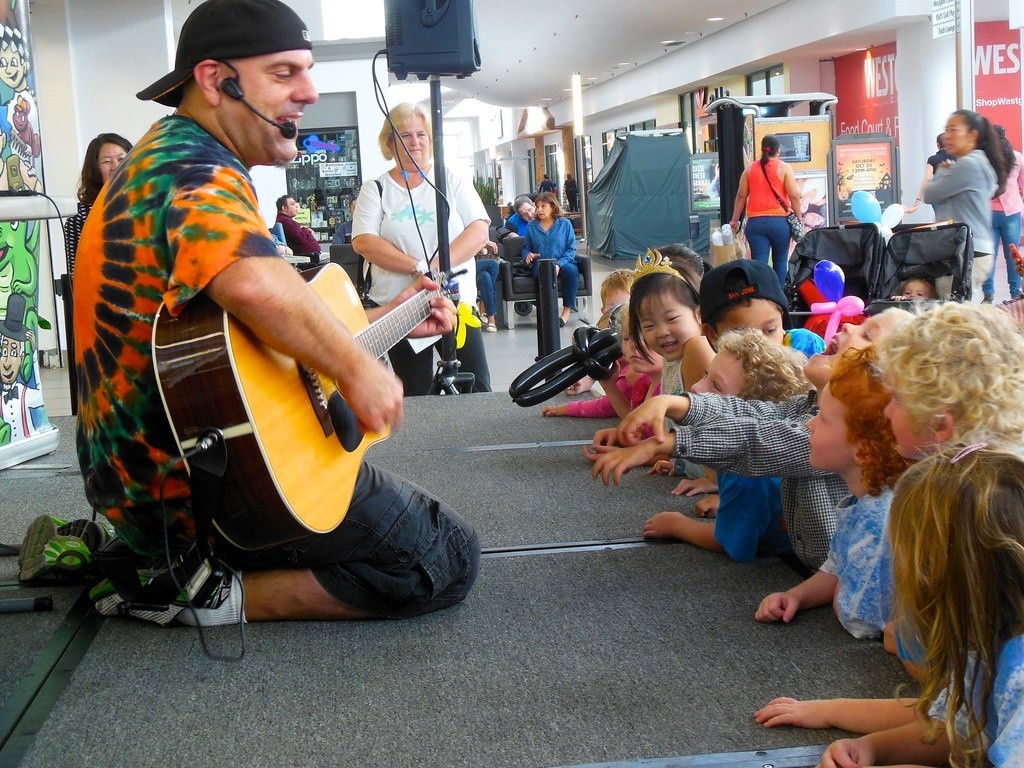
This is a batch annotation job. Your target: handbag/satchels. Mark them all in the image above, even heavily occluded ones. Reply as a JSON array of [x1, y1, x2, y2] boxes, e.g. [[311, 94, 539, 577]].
[[512, 259, 535, 276], [476, 251, 502, 281], [787, 212, 804, 242]]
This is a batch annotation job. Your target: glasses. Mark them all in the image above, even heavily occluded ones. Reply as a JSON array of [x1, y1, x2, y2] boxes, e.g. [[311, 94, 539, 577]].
[[518, 208, 533, 218]]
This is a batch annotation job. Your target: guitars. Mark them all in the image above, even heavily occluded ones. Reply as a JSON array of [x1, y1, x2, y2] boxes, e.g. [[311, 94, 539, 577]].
[[150, 261, 468, 551]]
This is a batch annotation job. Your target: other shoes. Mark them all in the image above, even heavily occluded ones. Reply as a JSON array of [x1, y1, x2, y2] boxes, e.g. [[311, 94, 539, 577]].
[[560, 315, 568, 327], [477, 302, 488, 324], [486, 324, 498, 332], [1002, 294, 1024, 305], [980, 296, 993, 304]]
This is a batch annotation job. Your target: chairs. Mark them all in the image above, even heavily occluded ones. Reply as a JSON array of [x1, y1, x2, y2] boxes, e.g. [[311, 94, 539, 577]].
[[480, 191, 593, 330], [329, 243, 372, 302]]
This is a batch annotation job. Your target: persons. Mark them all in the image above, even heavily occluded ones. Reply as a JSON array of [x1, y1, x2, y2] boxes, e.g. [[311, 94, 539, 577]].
[[335, 199, 379, 309], [65, 133, 131, 278], [19, 1, 479, 627], [475, 242, 501, 332], [272, 196, 320, 269], [351, 103, 493, 396], [503, 110, 1024, 767]]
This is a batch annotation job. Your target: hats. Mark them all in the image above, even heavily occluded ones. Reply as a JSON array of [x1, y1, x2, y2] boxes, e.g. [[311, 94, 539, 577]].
[[136, 0, 313, 108], [699, 258, 793, 353]]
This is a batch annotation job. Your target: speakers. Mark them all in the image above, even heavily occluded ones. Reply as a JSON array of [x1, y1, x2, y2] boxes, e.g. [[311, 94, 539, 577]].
[[384, 0, 482, 80]]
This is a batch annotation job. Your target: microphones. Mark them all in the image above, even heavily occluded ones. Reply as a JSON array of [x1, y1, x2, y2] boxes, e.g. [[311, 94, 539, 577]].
[[220, 77, 297, 139]]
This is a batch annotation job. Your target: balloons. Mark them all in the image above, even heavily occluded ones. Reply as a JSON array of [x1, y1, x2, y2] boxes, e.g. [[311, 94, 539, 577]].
[[881, 204, 904, 229], [811, 297, 865, 348], [509, 325, 623, 407], [815, 260, 844, 303], [851, 192, 882, 224]]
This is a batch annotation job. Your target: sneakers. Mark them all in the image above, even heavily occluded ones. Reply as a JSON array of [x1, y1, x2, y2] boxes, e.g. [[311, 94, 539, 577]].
[[17, 513, 115, 587], [89, 540, 214, 627]]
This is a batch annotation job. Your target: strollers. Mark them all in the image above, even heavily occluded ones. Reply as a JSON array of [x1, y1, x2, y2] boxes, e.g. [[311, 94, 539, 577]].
[[785, 220, 890, 335], [869, 222, 975, 335]]
